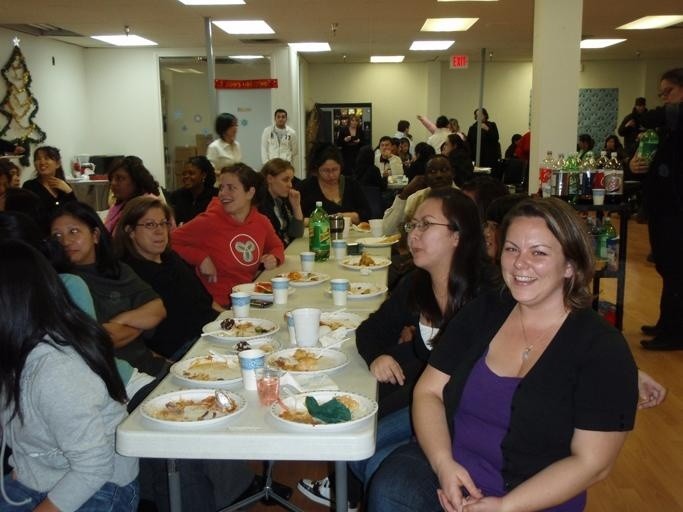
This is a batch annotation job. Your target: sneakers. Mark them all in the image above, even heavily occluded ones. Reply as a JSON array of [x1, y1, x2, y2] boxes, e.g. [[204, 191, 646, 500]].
[[296, 477, 357, 512], [235, 474, 292, 509]]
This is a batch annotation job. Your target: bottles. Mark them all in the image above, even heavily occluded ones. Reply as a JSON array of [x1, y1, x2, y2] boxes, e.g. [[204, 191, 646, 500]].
[[587, 217, 618, 262], [540, 151, 625, 205], [308, 202, 331, 261], [635, 128, 659, 163], [72, 156, 80, 177]]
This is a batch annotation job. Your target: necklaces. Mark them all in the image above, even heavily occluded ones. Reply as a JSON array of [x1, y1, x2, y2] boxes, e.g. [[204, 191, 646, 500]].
[[518, 308, 565, 361]]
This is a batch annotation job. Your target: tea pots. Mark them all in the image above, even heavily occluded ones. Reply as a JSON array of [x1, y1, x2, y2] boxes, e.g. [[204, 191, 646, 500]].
[[81, 161, 96, 177]]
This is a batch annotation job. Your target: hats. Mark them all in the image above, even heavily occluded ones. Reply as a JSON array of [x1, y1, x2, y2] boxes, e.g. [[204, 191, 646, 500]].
[[636, 97, 646, 105]]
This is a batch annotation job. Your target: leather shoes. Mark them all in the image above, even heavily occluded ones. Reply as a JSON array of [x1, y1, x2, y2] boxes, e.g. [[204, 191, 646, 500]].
[[640, 325, 683, 351]]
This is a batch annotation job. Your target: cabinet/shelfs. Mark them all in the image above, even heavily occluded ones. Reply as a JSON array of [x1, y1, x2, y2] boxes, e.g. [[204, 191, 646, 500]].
[[548, 197, 632, 329]]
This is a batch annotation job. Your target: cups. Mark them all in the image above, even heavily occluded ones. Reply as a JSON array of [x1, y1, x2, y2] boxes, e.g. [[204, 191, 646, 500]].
[[254, 368, 279, 405], [388, 176, 394, 184], [292, 308, 321, 347], [285, 311, 297, 345], [332, 279, 349, 306], [332, 240, 347, 261], [237, 350, 265, 391], [346, 242, 363, 257], [229, 292, 250, 319], [230, 216, 382, 406], [271, 277, 290, 305], [300, 252, 315, 272]]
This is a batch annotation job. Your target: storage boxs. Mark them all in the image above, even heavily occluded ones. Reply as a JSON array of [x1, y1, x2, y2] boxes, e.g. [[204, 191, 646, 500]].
[[175, 145, 196, 176]]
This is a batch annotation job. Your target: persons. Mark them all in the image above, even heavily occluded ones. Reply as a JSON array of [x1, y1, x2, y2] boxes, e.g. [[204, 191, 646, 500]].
[[629, 68, 683, 349], [105, 110, 530, 310], [0, 146, 77, 238], [369, 194, 638, 511], [578, 66, 659, 223], [1, 241, 142, 507], [0, 211, 157, 401], [295, 186, 667, 512], [50, 200, 293, 507], [355, 187, 517, 420]]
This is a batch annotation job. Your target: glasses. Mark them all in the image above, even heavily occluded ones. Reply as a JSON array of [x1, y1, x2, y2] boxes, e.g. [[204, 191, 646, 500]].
[[482, 223, 499, 232], [657, 86, 674, 97], [136, 222, 171, 228], [405, 219, 450, 231]]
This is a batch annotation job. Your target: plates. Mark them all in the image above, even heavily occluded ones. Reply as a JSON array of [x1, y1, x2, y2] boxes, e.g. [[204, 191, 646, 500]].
[[280, 272, 329, 287], [203, 318, 279, 341], [267, 348, 349, 374], [329, 283, 387, 298], [232, 283, 294, 299], [270, 391, 379, 427], [356, 238, 399, 247], [340, 255, 392, 269], [140, 388, 247, 426], [171, 355, 243, 384]]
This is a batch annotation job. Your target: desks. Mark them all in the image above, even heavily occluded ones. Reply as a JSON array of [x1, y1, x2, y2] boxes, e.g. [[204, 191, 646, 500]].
[[67, 179, 110, 211]]
